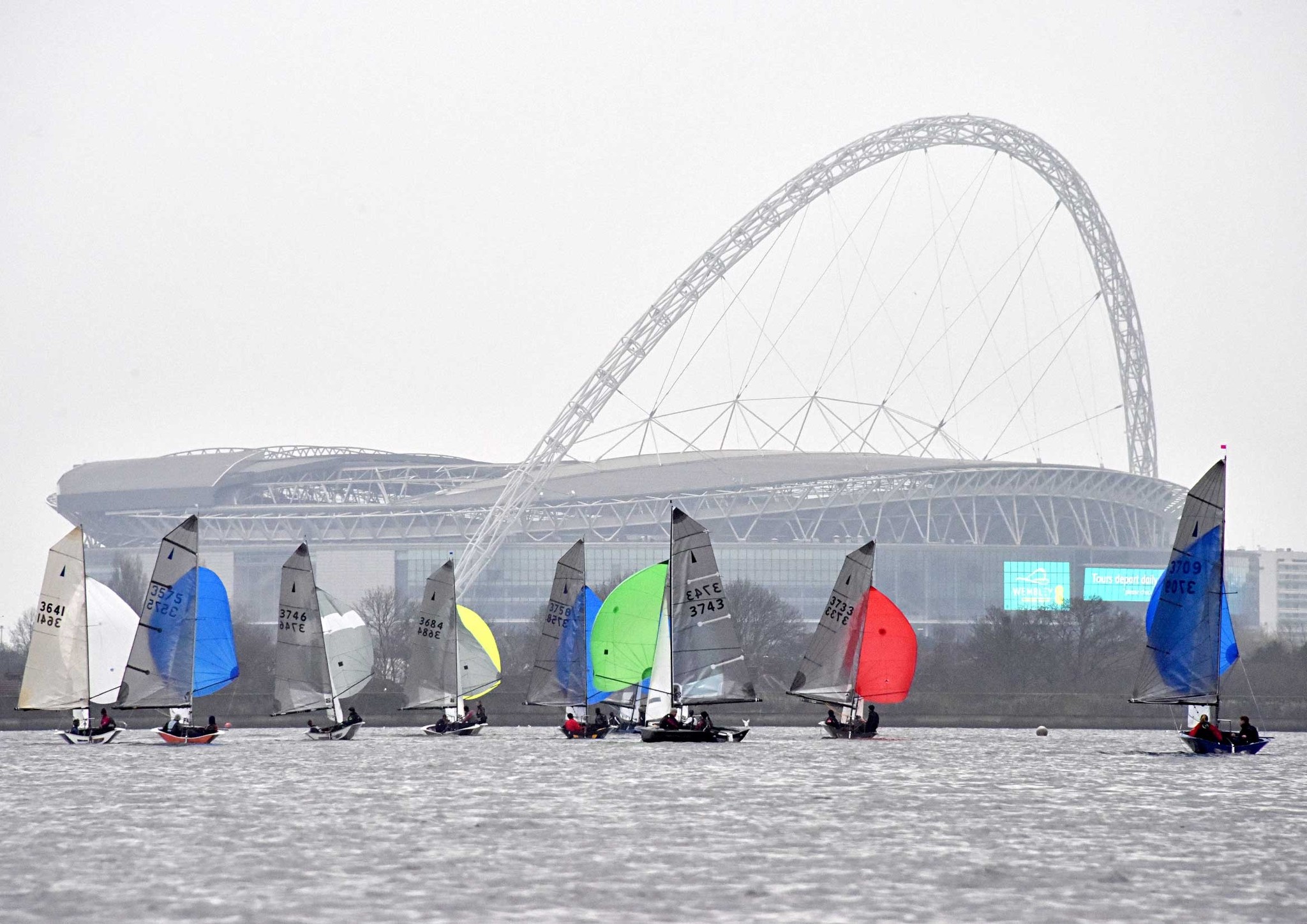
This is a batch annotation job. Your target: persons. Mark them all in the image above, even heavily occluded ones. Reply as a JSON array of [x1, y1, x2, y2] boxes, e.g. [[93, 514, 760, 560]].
[[99, 708, 116, 732], [435, 714, 450, 733], [1231, 716, 1259, 743], [476, 700, 486, 720], [1189, 714, 1222, 743], [683, 709, 697, 726], [609, 712, 621, 726], [694, 711, 712, 731], [825, 710, 849, 728], [204, 716, 218, 735], [855, 715, 863, 723], [334, 707, 362, 730], [308, 720, 331, 732], [458, 706, 485, 729], [595, 708, 608, 726], [163, 714, 181, 737], [659, 708, 680, 730], [565, 713, 586, 732], [863, 705, 879, 732], [70, 719, 80, 736]]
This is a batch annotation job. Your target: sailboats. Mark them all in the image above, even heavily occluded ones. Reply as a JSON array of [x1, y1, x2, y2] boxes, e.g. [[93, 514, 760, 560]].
[[393, 550, 505, 738], [524, 501, 762, 741], [787, 535, 916, 740], [15, 522, 141, 748], [113, 513, 238, 744], [1128, 452, 1267, 756], [270, 538, 374, 741]]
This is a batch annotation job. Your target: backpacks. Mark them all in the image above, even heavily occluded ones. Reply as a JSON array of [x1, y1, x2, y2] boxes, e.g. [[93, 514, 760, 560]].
[[659, 715, 669, 729], [873, 712, 879, 724], [436, 720, 445, 733]]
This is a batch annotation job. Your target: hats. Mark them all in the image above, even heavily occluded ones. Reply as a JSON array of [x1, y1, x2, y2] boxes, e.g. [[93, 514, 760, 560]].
[[868, 705, 875, 711], [174, 714, 181, 721], [101, 708, 106, 713], [567, 713, 574, 718], [595, 708, 600, 712]]
